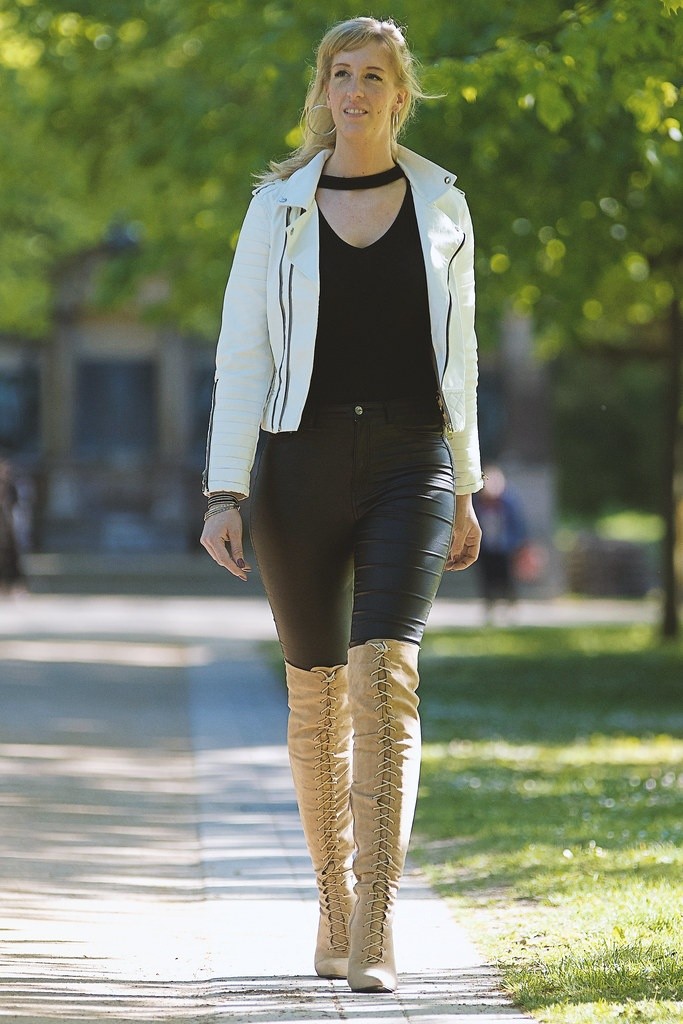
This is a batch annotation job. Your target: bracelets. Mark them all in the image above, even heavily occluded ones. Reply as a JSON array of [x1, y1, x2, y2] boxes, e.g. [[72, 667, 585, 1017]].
[[203, 493, 241, 522]]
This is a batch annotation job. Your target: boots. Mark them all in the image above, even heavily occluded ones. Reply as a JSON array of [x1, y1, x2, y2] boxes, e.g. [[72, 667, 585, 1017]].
[[284, 658, 356, 979], [344, 638, 423, 990]]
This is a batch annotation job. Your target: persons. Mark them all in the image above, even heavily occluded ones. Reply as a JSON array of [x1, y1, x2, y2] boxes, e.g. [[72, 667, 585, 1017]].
[[476, 464, 531, 605], [202, 17, 486, 992]]
